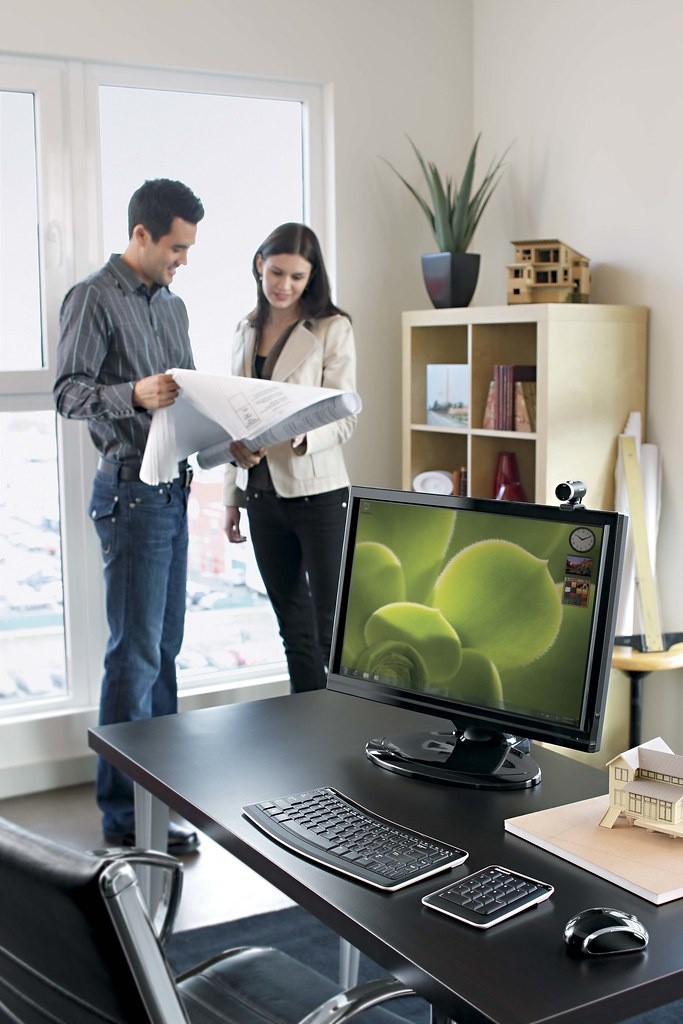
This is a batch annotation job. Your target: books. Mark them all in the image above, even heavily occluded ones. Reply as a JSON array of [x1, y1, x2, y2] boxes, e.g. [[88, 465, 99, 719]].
[[494, 363, 535, 431], [504, 793, 683, 906]]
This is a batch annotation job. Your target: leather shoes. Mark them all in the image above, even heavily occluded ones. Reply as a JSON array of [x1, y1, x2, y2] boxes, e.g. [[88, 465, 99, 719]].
[[105, 819, 199, 856]]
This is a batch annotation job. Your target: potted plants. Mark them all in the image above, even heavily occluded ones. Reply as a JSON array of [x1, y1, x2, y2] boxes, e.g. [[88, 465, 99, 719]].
[[379, 127, 518, 309]]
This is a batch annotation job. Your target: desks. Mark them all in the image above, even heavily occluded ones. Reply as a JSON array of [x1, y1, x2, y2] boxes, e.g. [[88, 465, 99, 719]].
[[88, 687, 683, 1024], [610, 642, 683, 749]]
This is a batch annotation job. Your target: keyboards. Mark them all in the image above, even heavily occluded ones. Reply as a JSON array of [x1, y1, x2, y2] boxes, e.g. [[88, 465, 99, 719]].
[[240, 786, 553, 929]]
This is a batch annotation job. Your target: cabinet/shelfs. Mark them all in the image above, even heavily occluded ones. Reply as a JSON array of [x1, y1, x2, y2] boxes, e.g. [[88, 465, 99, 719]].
[[401, 301, 649, 510]]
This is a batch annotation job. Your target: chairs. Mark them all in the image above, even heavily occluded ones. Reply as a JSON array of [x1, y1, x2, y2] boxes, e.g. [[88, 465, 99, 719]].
[[0, 817, 457, 1024]]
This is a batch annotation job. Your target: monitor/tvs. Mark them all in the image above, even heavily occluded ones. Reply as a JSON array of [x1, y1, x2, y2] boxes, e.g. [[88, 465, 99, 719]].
[[326, 485, 629, 790]]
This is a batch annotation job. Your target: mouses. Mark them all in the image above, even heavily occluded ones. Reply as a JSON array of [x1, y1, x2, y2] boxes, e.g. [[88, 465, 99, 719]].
[[563, 908, 649, 954]]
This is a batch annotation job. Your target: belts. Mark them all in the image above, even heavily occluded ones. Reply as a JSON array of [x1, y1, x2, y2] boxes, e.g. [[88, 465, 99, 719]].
[[98, 457, 195, 488]]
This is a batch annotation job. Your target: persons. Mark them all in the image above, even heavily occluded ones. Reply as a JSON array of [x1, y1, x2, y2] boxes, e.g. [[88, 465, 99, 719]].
[[51, 179, 266, 858], [224, 224, 358, 700]]
